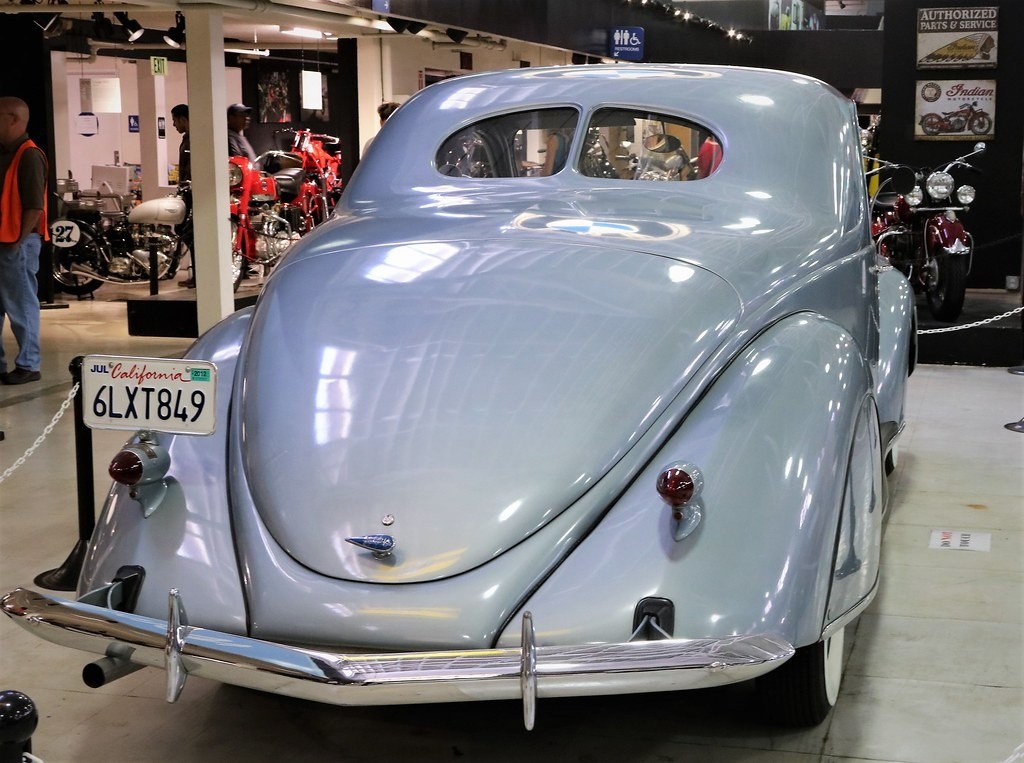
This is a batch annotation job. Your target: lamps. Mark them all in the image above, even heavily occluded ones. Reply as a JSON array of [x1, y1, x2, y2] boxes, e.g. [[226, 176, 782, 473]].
[[20, 0, 68, 30], [163, 11, 186, 48], [839, 0, 847, 10], [90, 0, 145, 42]]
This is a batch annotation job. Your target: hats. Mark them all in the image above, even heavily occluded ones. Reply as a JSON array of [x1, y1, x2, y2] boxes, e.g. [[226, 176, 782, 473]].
[[228, 103, 252, 115]]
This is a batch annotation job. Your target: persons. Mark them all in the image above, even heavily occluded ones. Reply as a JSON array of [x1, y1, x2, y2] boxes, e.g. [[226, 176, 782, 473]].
[[363, 102, 401, 157], [170, 104, 196, 288], [542, 128, 575, 176], [697, 135, 722, 180], [130, 169, 142, 200], [227, 104, 260, 170], [0, 97, 51, 386]]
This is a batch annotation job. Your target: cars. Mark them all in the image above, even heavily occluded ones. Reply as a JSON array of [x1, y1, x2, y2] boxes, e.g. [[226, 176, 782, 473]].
[[1, 62, 921, 730]]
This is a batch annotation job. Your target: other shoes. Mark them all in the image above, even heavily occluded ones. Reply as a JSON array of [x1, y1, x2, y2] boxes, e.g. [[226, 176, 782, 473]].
[[179, 278, 195, 288], [1, 368, 41, 384], [0, 372, 8, 382]]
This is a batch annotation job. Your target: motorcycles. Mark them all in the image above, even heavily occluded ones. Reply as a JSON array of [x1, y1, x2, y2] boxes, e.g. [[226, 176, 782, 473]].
[[614, 118, 701, 182], [47, 127, 192, 306], [225, 126, 347, 292], [917, 100, 993, 135], [536, 124, 618, 180], [856, 126, 986, 324]]
